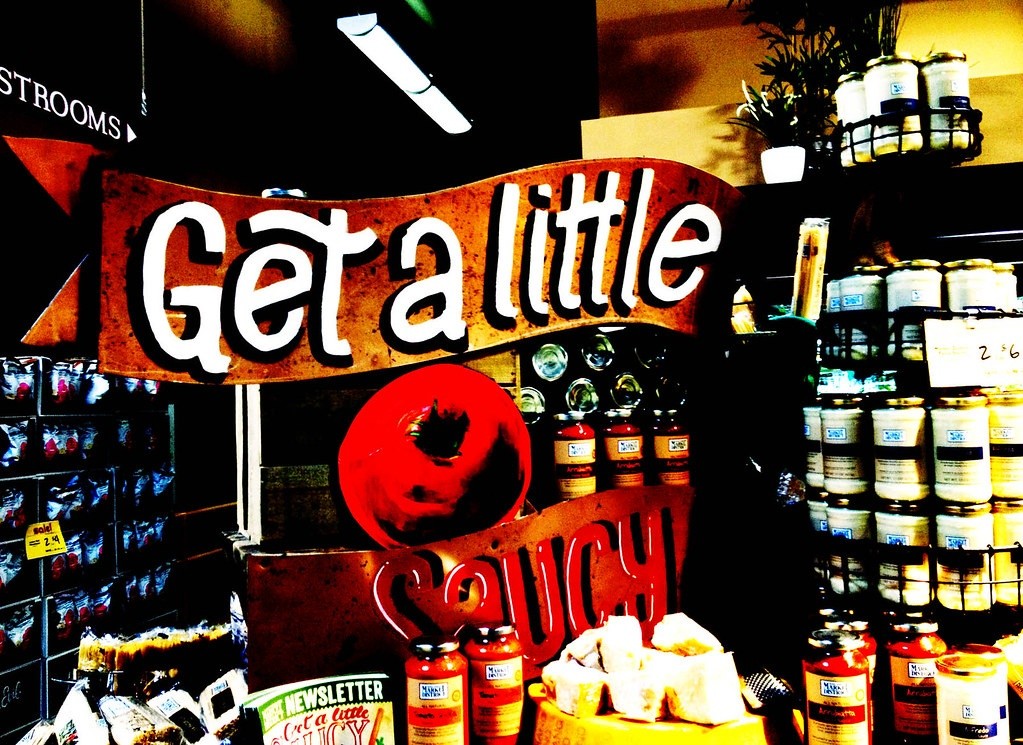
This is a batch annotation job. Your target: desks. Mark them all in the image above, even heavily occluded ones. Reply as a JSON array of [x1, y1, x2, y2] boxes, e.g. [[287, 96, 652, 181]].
[[528, 675, 804, 745]]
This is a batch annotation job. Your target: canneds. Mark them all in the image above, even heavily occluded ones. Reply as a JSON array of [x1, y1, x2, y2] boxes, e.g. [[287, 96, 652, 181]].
[[801, 258, 1023, 745], [834, 50, 972, 167]]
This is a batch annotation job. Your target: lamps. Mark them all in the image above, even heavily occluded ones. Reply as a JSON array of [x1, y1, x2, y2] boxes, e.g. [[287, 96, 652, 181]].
[[337, 0, 474, 134]]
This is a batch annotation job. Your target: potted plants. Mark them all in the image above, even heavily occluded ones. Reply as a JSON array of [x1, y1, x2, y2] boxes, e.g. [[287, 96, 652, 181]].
[[722, 0, 909, 183]]
